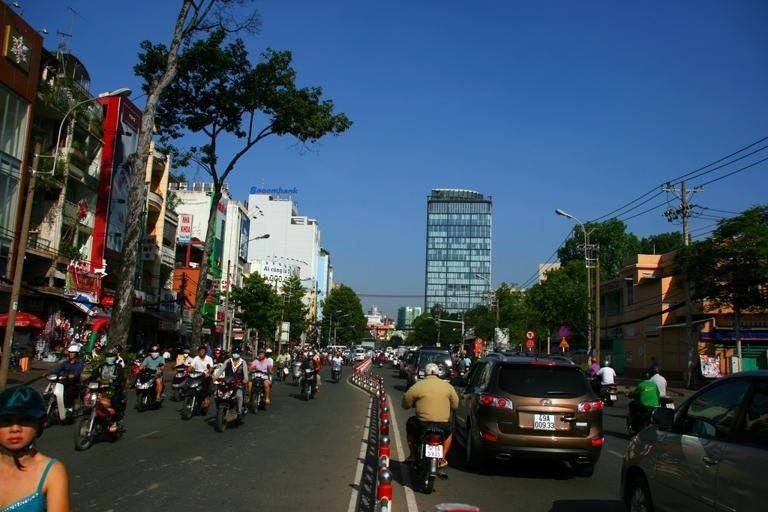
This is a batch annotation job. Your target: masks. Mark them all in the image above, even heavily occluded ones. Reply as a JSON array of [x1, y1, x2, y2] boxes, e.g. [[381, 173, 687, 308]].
[[149, 353, 160, 359], [233, 354, 240, 359], [105, 357, 117, 364]]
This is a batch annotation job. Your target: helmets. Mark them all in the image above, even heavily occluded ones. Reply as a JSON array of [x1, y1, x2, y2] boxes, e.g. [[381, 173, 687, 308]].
[[69, 345, 343, 355], [592, 357, 596, 363], [425, 363, 439, 375], [640, 365, 659, 379], [0, 385, 46, 416], [603, 360, 610, 365]]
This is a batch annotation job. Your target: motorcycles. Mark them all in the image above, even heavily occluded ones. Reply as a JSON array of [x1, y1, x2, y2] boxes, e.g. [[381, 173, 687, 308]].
[[129, 346, 348, 433], [621, 389, 650, 437], [373, 351, 385, 368], [456, 370, 464, 377], [590, 378, 617, 406], [73, 379, 127, 451], [657, 395, 674, 410], [464, 366, 469, 371], [39, 372, 85, 433], [404, 423, 450, 494]]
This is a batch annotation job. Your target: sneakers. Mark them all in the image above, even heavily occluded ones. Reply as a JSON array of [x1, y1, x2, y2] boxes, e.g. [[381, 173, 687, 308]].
[[440, 460, 448, 468], [406, 456, 417, 462]]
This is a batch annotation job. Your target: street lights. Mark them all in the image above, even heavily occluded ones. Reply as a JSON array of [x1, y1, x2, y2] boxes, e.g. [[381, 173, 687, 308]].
[[325, 307, 356, 348], [448, 297, 465, 336], [0, 84, 132, 399], [473, 274, 500, 331], [222, 233, 270, 349], [553, 206, 592, 365], [275, 276, 312, 357], [427, 316, 440, 342]]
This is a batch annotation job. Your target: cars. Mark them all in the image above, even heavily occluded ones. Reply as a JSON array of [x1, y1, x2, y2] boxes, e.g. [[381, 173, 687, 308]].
[[343, 346, 372, 363], [384, 345, 416, 379], [614, 368, 766, 512]]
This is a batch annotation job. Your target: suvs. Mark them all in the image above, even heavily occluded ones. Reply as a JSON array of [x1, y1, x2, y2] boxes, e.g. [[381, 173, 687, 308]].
[[406, 346, 454, 392], [449, 348, 605, 475]]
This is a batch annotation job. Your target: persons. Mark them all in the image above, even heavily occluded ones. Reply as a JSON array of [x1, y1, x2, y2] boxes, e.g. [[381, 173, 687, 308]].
[[400, 361, 460, 469], [373, 348, 386, 368], [2, 342, 166, 432], [163, 344, 355, 416], [451, 340, 473, 379], [584, 356, 676, 438], [1, 385, 70, 510]]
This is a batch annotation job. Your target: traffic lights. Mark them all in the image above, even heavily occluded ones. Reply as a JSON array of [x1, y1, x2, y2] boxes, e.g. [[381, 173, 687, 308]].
[[435, 315, 438, 326]]
